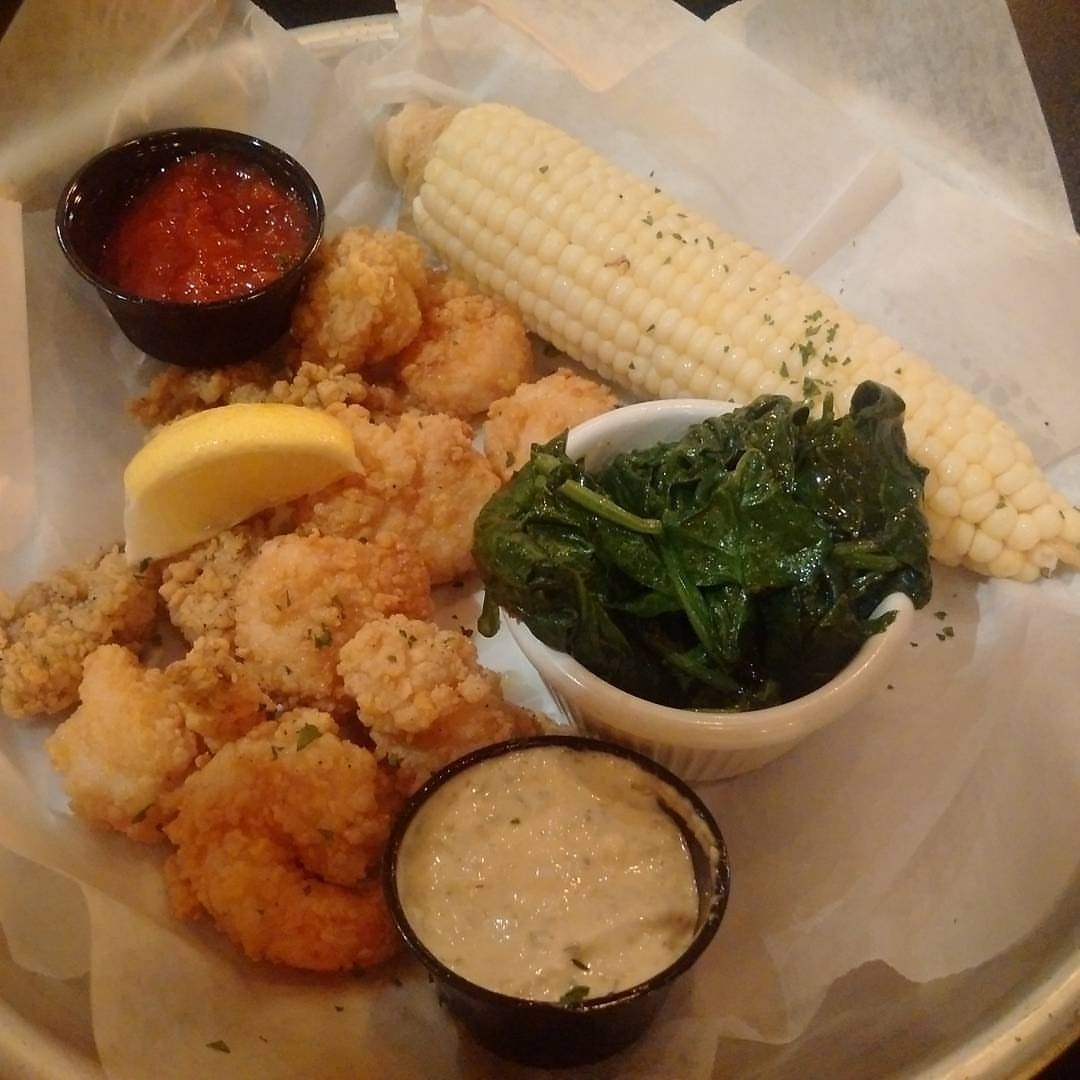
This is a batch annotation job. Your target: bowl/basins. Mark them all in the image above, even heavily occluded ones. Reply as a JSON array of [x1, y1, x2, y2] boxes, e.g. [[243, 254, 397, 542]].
[[53, 127, 326, 371], [376, 730, 733, 1070], [498, 395, 915, 786]]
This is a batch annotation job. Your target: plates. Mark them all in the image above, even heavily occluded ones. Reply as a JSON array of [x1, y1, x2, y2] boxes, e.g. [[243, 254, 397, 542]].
[[0, 10, 1080, 1080]]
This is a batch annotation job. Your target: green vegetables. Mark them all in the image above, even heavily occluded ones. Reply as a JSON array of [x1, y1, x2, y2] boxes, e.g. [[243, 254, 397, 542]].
[[470, 376, 935, 714]]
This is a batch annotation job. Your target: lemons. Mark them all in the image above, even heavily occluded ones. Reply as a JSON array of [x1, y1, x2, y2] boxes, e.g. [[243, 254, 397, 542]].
[[122, 405, 365, 567]]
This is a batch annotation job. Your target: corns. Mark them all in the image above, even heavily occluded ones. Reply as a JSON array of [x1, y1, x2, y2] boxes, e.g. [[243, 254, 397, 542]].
[[375, 99, 1079, 586]]
